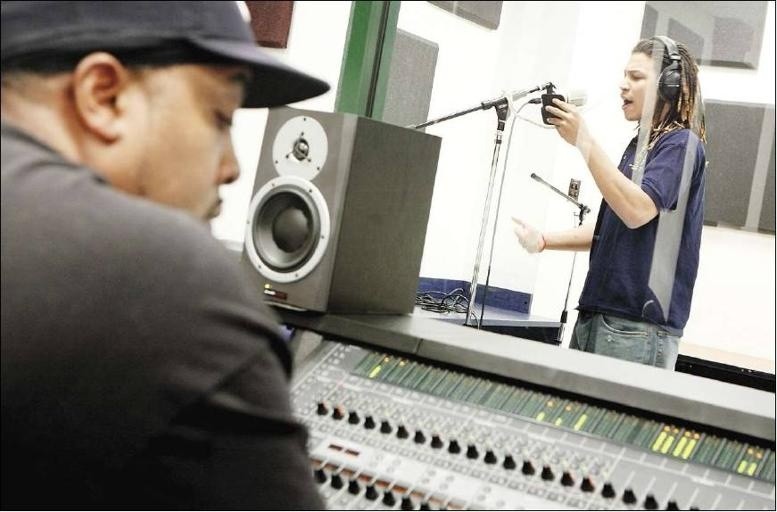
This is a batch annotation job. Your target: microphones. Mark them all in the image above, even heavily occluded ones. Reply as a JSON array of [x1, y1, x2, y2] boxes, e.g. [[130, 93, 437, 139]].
[[529, 91, 587, 106]]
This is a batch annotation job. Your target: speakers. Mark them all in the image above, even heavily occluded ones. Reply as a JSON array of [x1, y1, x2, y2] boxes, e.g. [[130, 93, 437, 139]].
[[240, 106, 442, 316]]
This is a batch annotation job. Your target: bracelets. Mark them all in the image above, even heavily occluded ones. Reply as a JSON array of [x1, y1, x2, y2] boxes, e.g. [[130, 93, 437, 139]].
[[539, 235, 546, 253]]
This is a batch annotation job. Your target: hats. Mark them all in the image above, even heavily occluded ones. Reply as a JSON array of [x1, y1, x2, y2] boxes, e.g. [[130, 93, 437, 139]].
[[2, 2, 330, 107]]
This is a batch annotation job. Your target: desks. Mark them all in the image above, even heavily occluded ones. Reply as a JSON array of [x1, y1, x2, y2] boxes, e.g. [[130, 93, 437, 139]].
[[414, 300, 563, 343]]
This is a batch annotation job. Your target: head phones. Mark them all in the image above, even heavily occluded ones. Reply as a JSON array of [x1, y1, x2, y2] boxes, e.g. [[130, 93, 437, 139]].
[[651, 36, 682, 103]]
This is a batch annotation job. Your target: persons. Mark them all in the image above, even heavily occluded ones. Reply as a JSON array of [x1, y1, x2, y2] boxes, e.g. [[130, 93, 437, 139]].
[[0, 2, 332, 508], [513, 36, 705, 369]]
